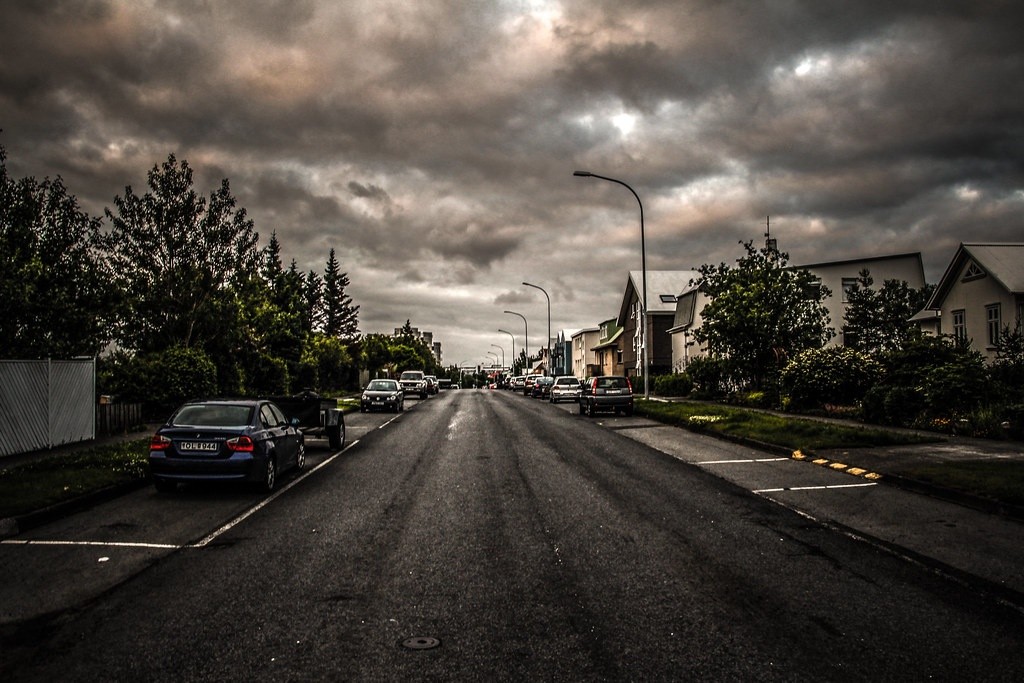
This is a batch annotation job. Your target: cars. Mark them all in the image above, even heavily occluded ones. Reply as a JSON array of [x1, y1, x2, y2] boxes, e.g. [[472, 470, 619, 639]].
[[480, 374, 525, 392], [550, 376, 583, 404], [147, 396, 307, 496], [424, 374, 439, 395], [532, 376, 555, 400], [361, 378, 403, 412]]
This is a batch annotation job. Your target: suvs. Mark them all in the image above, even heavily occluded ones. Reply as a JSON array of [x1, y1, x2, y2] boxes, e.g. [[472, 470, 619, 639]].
[[576, 375, 633, 419], [523, 374, 543, 395], [398, 370, 428, 400]]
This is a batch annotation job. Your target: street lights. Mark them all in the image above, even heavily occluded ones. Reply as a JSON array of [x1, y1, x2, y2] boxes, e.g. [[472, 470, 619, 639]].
[[488, 351, 498, 371], [570, 168, 651, 404], [498, 329, 515, 376], [503, 310, 528, 374], [522, 282, 551, 379], [491, 344, 504, 372]]
